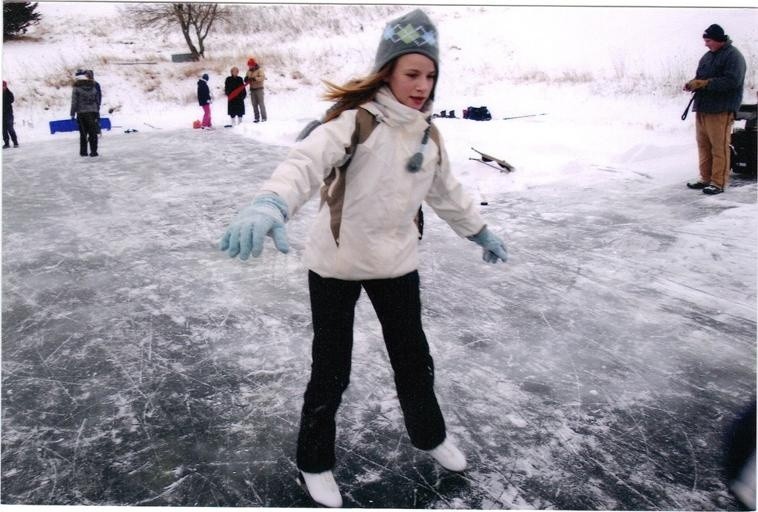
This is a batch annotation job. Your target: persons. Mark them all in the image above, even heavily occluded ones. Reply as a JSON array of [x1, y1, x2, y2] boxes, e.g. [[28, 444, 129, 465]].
[[685, 24, 746, 195], [221, 10, 506, 508], [225, 67, 246, 125], [244, 59, 267, 123], [2, 81, 18, 148], [70, 75, 99, 156], [86, 70, 101, 142], [197, 74, 214, 129]]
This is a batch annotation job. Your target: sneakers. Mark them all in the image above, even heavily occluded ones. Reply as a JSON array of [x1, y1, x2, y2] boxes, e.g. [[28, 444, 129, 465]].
[[687, 183, 723, 194], [425, 438, 466, 472], [302, 470, 343, 508]]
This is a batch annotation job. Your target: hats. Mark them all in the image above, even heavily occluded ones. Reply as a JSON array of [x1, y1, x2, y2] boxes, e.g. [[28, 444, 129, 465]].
[[703, 24, 724, 41], [376, 9, 438, 72], [203, 74, 209, 81], [248, 58, 257, 66]]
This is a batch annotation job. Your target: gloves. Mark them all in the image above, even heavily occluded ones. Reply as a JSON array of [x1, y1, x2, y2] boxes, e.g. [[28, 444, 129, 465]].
[[469, 226, 507, 264], [220, 194, 288, 260], [688, 80, 708, 92]]
